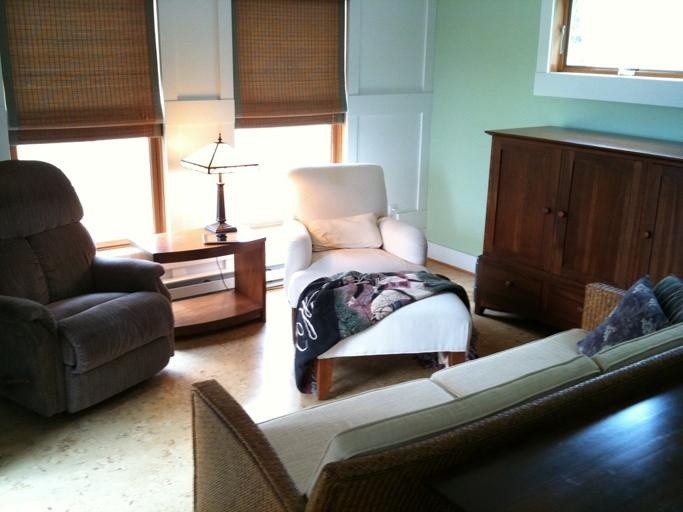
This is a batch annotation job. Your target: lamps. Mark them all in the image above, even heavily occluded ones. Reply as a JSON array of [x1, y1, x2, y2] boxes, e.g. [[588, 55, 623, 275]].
[[179, 133, 261, 234]]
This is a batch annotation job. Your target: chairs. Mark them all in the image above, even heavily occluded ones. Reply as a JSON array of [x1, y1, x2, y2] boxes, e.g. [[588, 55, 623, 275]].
[[283, 164, 473, 400], [0, 160, 174, 418]]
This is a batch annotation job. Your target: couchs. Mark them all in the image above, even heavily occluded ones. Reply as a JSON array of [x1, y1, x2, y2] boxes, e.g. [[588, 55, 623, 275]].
[[191, 281, 683, 512]]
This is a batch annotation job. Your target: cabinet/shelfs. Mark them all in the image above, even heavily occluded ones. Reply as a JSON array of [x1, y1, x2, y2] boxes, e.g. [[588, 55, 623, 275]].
[[633, 138, 683, 288], [475, 126, 642, 330]]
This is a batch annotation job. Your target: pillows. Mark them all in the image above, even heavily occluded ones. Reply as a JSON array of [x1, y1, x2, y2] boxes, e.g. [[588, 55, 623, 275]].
[[653, 274, 683, 324], [576, 275, 670, 356], [307, 213, 383, 252]]
